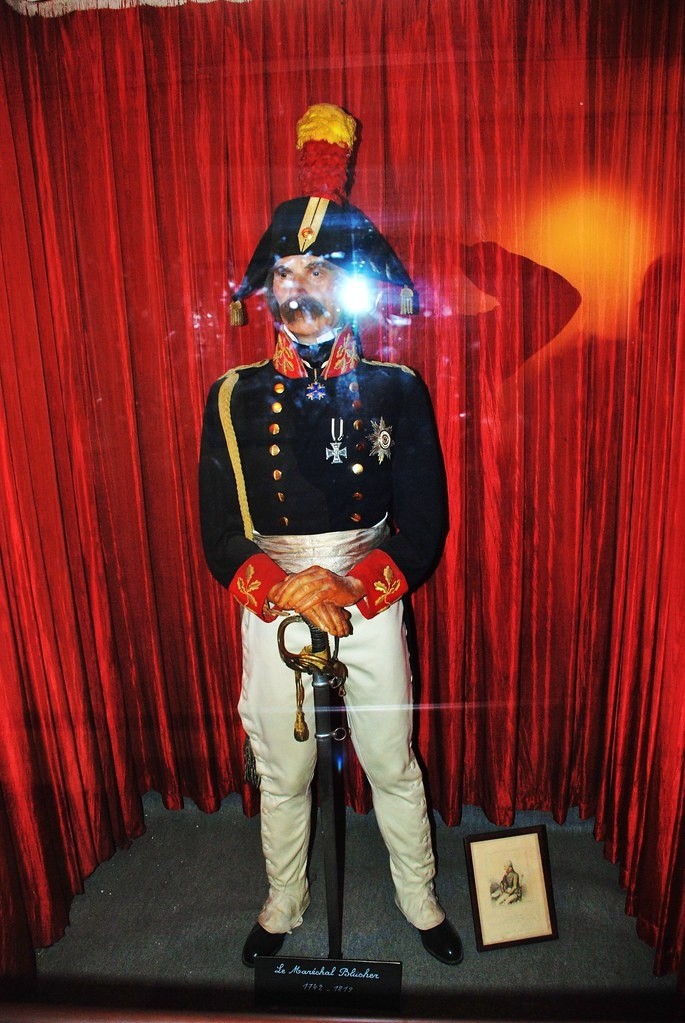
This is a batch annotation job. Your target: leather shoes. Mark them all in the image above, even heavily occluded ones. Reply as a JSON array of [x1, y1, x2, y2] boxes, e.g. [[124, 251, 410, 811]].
[[242, 922, 287, 969], [418, 918, 464, 965]]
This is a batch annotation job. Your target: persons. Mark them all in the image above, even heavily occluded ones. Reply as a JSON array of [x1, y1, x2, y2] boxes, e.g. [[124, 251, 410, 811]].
[[196, 101, 463, 964], [497, 861, 522, 905]]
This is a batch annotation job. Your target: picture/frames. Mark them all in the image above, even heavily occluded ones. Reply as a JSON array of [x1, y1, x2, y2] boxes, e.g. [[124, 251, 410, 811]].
[[464, 824, 559, 954]]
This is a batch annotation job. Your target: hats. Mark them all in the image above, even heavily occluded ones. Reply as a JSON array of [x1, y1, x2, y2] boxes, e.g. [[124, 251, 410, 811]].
[[228, 196, 415, 326]]
